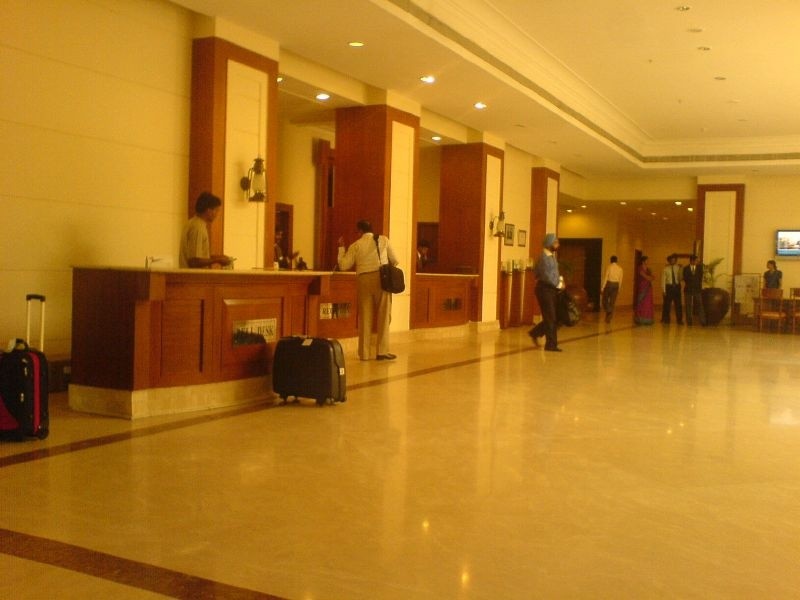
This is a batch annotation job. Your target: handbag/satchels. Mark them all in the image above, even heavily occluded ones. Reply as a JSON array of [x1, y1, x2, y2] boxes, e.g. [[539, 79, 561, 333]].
[[556, 291, 581, 326], [379, 264, 405, 293]]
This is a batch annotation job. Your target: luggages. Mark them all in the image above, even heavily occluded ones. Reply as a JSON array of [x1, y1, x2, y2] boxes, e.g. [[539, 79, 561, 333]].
[[0, 294, 50, 441], [272, 335, 347, 407]]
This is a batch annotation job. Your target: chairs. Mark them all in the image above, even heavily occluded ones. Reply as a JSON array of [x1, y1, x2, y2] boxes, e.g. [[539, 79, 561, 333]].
[[756, 287, 800, 335]]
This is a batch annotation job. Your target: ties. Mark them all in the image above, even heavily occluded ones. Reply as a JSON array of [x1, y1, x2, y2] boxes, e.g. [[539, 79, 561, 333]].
[[672, 265, 675, 288]]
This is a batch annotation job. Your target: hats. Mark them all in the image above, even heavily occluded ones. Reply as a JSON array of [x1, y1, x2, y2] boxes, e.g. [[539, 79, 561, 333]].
[[542, 234, 557, 249]]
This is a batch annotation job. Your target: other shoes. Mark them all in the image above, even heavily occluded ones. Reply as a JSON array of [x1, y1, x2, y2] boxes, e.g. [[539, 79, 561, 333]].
[[528, 330, 541, 347], [544, 346, 562, 351], [376, 353, 396, 360]]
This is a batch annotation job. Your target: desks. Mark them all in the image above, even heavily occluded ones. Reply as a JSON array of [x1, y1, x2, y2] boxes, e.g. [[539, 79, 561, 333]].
[[751, 295, 799, 328]]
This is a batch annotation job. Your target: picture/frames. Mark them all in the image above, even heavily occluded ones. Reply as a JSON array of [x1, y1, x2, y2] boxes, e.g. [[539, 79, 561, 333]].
[[518, 230, 526, 246], [503, 224, 515, 246]]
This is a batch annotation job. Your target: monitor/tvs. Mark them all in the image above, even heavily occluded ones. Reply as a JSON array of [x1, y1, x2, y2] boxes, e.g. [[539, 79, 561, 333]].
[[776, 230, 800, 256]]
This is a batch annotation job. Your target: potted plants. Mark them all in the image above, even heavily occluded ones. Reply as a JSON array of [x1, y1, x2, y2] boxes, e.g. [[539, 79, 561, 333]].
[[695, 257, 731, 325]]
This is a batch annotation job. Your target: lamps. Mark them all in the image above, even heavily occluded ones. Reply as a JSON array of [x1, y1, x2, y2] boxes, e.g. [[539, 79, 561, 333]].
[[240, 157, 270, 203], [489, 212, 506, 236]]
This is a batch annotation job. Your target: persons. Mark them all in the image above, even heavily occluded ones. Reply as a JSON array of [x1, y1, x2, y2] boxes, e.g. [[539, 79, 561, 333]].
[[529, 234, 565, 351], [600, 255, 623, 323], [416, 239, 430, 274], [683, 255, 706, 327], [763, 260, 783, 289], [274, 227, 290, 268], [338, 220, 396, 362], [178, 192, 231, 269], [661, 257, 685, 324], [635, 256, 655, 324]]
